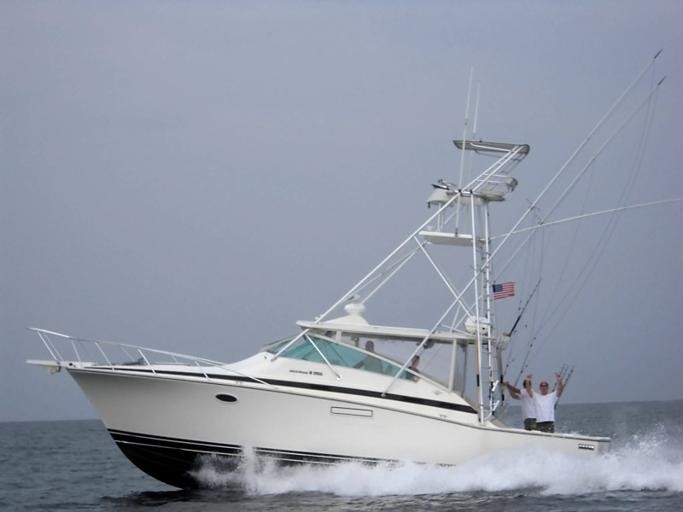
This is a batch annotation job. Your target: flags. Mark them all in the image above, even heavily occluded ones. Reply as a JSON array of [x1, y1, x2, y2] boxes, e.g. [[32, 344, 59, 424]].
[[493, 282, 514, 299]]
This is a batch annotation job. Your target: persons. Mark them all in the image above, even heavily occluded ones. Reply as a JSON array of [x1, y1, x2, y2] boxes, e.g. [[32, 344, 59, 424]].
[[526, 374, 563, 433], [504, 380, 536, 430], [353, 341, 384, 374], [405, 355, 420, 381]]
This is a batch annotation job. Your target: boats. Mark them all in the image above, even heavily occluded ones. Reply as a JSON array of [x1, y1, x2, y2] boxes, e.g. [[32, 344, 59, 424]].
[[24, 46, 668, 490]]
